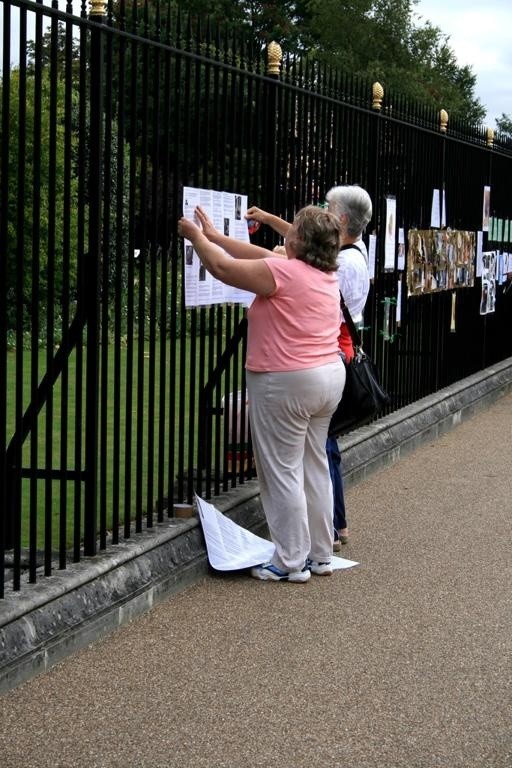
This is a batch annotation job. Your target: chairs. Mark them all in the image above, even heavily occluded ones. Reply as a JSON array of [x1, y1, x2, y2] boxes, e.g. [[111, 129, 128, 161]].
[[334, 534, 348, 551], [252, 559, 311, 583], [306, 558, 332, 576]]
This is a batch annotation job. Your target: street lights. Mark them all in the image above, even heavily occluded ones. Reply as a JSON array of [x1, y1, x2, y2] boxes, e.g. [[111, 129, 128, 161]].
[[340, 345, 389, 422]]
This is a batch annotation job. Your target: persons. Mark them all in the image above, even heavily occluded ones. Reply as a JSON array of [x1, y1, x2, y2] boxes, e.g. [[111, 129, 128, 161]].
[[177, 202, 348, 584], [243, 184, 375, 552]]
